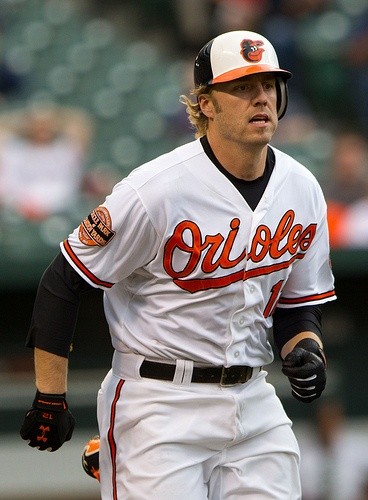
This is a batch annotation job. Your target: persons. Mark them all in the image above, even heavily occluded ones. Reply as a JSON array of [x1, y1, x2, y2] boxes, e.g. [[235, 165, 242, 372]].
[[20, 30, 337, 500], [0, 98, 368, 500]]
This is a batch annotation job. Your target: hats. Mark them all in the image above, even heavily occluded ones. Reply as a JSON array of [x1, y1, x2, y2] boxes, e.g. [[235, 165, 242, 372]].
[[193, 30, 294, 88]]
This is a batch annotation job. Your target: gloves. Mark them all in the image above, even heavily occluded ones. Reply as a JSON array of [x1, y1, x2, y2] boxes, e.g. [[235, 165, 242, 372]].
[[279, 337, 327, 404], [21, 390, 77, 455]]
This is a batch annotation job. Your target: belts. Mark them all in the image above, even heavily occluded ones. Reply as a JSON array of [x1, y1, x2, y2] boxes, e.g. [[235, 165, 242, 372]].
[[141, 358, 250, 385]]
